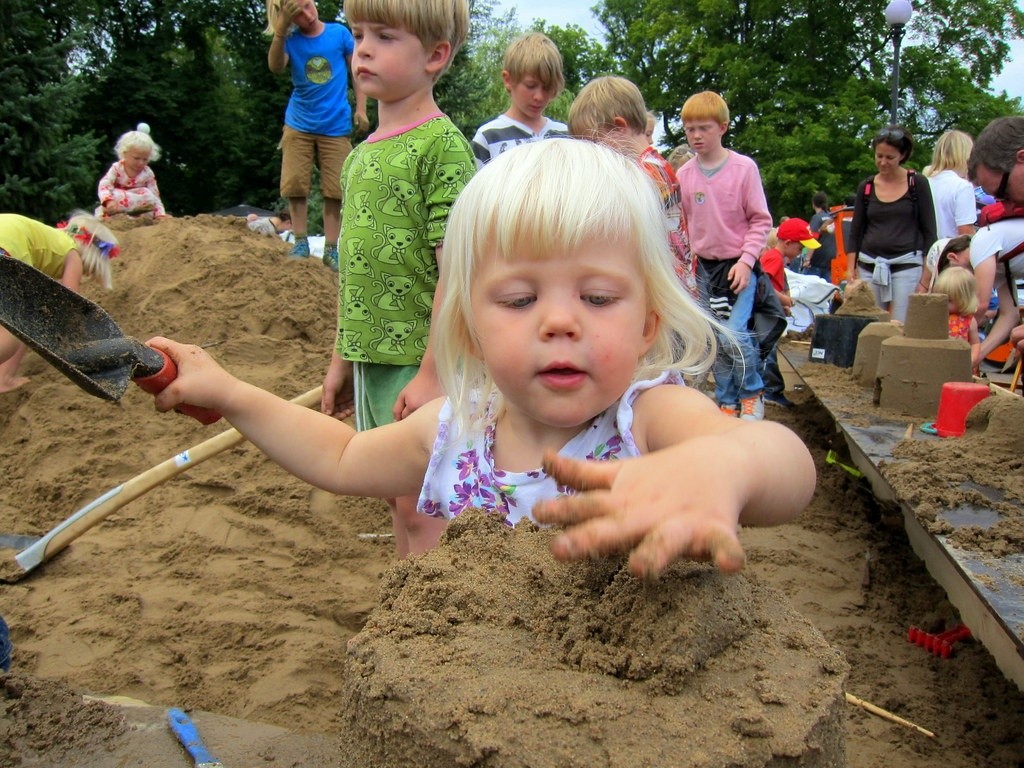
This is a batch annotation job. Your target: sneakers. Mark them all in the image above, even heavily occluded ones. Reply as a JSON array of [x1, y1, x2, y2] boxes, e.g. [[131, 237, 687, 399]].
[[740, 396, 765, 421], [762, 392, 792, 409], [323, 249, 339, 273], [720, 402, 738, 418], [293, 242, 310, 259]]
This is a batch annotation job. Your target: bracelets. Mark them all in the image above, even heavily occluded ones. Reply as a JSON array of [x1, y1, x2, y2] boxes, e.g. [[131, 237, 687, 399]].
[[919, 280, 929, 290]]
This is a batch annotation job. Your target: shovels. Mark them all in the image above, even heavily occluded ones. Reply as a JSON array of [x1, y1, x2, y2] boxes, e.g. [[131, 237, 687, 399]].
[[169, 705, 222, 768], [1, 379, 330, 586], [0, 248, 225, 426]]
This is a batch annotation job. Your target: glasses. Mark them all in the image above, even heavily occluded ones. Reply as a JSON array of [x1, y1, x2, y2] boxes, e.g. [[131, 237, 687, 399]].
[[879, 129, 905, 141], [993, 172, 1010, 199]]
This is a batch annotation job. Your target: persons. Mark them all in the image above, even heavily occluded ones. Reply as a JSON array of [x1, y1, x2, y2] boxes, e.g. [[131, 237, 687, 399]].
[[922, 130, 977, 241], [645, 112, 696, 175], [247, 209, 292, 236], [846, 125, 938, 324], [768, 192, 856, 283], [470, 32, 569, 167], [675, 91, 773, 421], [0, 209, 120, 393], [567, 76, 700, 380], [926, 217, 1024, 369], [146, 138, 817, 578], [933, 266, 980, 377], [967, 116, 1024, 397], [95, 131, 173, 221], [261, 0, 370, 272], [322, 0, 478, 561], [759, 218, 822, 408]]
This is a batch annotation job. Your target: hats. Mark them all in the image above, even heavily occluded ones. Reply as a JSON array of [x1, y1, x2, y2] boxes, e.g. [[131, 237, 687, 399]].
[[926, 237, 952, 293], [777, 218, 822, 249]]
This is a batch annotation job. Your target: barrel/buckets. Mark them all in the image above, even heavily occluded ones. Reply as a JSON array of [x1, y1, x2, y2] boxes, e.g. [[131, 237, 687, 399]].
[[921, 382, 991, 438]]
